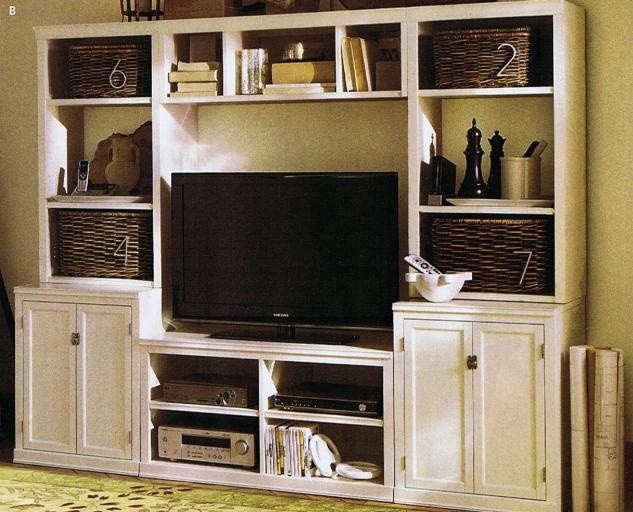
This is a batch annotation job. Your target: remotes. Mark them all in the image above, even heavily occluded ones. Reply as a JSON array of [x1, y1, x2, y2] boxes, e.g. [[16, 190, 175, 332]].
[[403, 254, 443, 275]]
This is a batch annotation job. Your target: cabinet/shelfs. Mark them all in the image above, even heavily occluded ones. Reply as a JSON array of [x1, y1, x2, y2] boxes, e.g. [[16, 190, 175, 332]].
[[147, 350, 385, 487], [44, 34, 196, 282], [12, 286, 161, 474], [391, 304, 587, 507], [417, 14, 587, 304], [160, 8, 407, 105]]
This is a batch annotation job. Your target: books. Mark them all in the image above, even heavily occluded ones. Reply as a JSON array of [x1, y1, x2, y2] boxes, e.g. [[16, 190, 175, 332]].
[[261, 423, 323, 478], [166, 32, 401, 99]]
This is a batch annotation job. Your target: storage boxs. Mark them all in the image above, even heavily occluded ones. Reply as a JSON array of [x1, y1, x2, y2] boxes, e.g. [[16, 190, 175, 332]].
[[163, 1, 236, 18]]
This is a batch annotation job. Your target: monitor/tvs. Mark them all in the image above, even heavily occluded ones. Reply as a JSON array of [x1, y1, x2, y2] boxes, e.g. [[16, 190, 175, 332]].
[[171, 172, 400, 344]]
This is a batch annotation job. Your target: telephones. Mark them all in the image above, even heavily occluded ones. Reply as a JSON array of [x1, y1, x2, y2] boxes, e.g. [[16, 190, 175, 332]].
[[70, 159, 120, 197]]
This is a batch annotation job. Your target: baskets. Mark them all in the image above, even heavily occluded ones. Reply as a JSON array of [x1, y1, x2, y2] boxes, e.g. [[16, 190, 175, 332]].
[[431, 217, 553, 295], [67, 42, 150, 99], [432, 26, 544, 88], [56, 210, 152, 279]]
